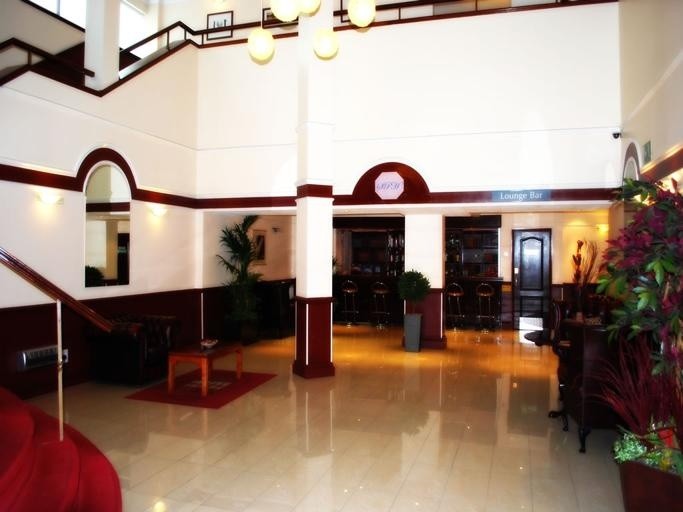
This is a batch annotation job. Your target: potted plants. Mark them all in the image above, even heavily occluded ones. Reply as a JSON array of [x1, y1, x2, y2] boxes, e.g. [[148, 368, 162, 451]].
[[217, 216, 266, 345], [396, 270, 431, 353]]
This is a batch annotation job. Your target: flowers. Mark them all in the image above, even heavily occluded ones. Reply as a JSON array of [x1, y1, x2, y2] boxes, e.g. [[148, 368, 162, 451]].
[[596, 176, 683, 467], [568, 237, 598, 299]]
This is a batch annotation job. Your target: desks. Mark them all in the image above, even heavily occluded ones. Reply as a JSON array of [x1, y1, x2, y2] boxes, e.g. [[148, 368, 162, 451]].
[[165, 341, 244, 397]]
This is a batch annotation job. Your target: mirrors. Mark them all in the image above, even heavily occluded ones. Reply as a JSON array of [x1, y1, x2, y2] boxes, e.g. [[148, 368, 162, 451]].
[[83, 161, 131, 286]]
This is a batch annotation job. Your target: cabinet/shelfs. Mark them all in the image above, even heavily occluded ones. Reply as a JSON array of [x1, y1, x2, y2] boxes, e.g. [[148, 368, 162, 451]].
[[349, 230, 403, 275], [552, 318, 639, 452], [445, 229, 499, 281]]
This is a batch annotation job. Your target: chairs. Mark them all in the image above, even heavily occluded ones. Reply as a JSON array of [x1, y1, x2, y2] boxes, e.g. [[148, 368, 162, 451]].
[[87, 312, 177, 386], [337, 277, 498, 332]]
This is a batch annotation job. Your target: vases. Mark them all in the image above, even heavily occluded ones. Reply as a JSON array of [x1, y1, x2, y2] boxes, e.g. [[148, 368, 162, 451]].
[[611, 446, 682, 512]]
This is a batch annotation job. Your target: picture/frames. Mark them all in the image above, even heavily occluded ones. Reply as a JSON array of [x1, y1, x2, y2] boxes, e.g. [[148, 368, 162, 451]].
[[250, 227, 268, 266], [261, 7, 277, 22], [205, 9, 235, 41]]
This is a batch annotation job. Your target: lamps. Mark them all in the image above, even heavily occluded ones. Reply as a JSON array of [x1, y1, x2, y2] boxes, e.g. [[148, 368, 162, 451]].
[[244, 0, 376, 63]]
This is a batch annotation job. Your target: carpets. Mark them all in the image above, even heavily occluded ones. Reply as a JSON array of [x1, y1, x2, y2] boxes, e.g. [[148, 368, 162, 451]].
[[523, 330, 552, 347], [124, 364, 277, 412]]
